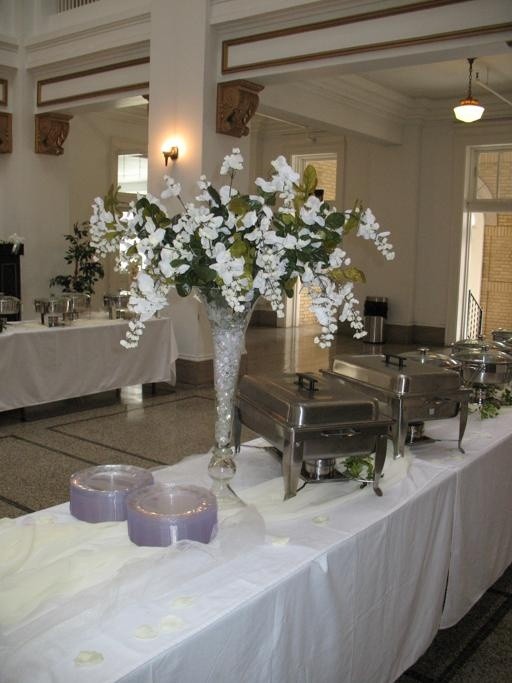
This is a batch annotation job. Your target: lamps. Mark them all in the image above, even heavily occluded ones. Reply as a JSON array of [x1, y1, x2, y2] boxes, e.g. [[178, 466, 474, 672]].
[[162, 145, 178, 166], [453, 56, 486, 121]]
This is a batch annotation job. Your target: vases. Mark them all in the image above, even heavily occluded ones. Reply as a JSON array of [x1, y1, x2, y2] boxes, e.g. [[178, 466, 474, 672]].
[[187, 286, 262, 478]]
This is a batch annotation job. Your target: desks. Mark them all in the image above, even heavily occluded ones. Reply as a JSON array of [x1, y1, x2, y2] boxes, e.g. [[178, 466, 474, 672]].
[[0, 397, 512, 683], [0, 309, 177, 410]]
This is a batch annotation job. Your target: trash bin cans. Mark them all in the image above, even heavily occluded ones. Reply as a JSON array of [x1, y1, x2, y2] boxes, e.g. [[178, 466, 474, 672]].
[[361, 295, 389, 344]]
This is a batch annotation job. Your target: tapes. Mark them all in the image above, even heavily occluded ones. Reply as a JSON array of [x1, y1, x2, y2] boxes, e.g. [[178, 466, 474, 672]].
[[125, 481, 217, 549], [68, 463, 155, 523]]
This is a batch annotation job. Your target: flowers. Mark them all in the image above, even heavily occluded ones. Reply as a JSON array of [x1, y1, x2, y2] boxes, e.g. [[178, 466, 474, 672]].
[[92, 150, 393, 350]]
